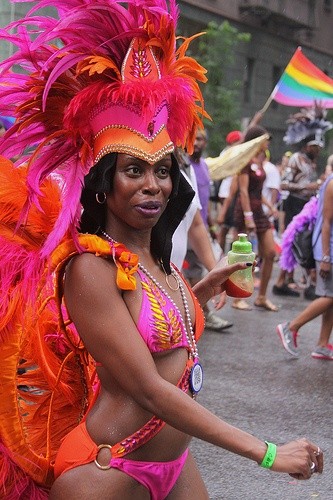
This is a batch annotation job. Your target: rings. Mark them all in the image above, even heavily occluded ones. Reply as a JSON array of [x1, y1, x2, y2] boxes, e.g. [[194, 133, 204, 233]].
[[311, 462, 316, 469], [315, 447, 320, 456]]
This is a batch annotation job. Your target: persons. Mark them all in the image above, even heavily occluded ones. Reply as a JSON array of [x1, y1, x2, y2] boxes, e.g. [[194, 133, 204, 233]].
[[165, 115, 333, 330], [277, 173, 333, 361], [0, 1, 324, 500]]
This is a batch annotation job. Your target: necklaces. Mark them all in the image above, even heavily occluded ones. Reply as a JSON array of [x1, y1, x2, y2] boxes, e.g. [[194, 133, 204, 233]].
[[101, 227, 199, 357]]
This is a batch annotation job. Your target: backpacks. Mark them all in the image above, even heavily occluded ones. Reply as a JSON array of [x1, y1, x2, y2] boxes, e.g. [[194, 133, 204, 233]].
[[291, 217, 322, 268]]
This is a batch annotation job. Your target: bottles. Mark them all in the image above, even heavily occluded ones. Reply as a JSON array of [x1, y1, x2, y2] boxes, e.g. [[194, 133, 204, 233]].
[[227, 233, 256, 298]]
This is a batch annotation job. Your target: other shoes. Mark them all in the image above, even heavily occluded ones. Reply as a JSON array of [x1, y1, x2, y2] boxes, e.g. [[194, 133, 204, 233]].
[[301, 277, 306, 283], [288, 283, 298, 288], [254, 302, 278, 312], [231, 302, 253, 311]]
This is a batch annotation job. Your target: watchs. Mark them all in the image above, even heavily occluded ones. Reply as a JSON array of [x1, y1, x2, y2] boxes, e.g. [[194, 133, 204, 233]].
[[322, 256, 330, 263]]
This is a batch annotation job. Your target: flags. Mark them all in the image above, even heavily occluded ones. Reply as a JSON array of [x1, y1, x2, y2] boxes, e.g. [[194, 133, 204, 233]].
[[273, 48, 333, 108]]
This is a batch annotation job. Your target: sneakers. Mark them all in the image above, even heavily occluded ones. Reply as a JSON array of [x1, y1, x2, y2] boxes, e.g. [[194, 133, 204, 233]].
[[312, 344, 333, 360], [276, 322, 298, 355], [204, 312, 233, 330], [272, 284, 300, 296], [305, 286, 320, 300]]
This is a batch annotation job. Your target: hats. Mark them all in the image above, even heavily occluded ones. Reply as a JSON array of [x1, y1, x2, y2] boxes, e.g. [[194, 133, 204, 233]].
[[0, 0, 212, 257], [226, 131, 241, 143]]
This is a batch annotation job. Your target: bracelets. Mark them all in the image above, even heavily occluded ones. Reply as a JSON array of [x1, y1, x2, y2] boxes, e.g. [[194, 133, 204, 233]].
[[259, 441, 276, 469]]
